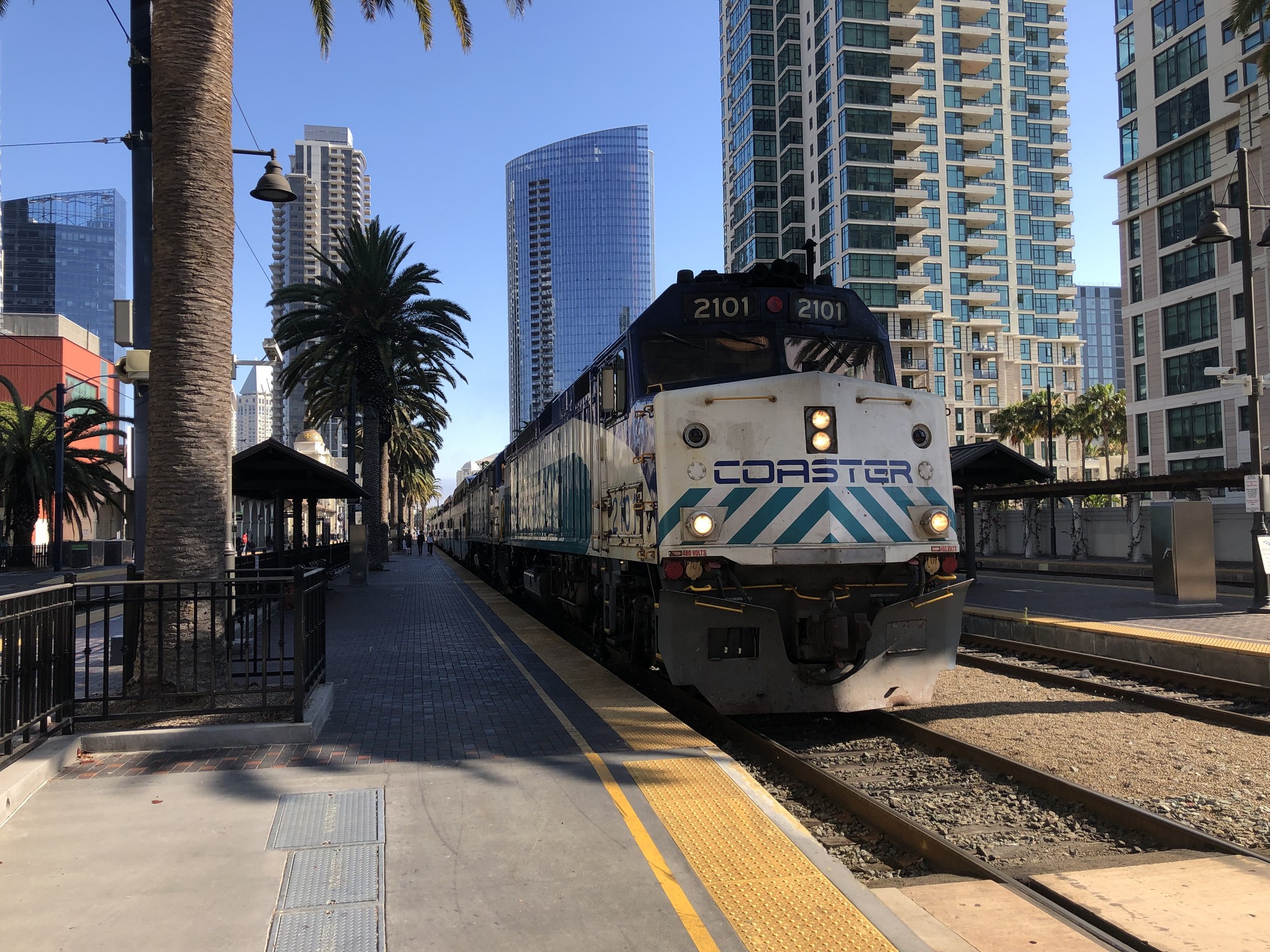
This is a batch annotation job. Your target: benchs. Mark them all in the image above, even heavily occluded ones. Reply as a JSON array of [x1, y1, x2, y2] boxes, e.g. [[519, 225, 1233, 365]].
[[291, 559, 340, 591]]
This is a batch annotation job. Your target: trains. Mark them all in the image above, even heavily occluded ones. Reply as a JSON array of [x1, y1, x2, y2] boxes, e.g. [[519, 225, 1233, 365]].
[[424, 259, 980, 719]]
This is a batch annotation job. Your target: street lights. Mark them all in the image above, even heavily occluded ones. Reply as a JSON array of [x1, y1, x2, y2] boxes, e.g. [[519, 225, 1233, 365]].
[[1193, 146, 1270, 611], [1030, 383, 1073, 560], [53, 373, 120, 574]]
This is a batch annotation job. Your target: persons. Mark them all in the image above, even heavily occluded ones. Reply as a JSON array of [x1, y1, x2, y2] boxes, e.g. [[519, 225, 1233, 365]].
[[240, 531, 319, 554], [405, 530, 434, 555]]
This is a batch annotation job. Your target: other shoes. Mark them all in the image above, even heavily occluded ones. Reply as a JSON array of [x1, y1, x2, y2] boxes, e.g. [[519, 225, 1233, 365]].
[[410, 553, 412, 554], [427, 552, 432, 555], [407, 553, 409, 554]]
